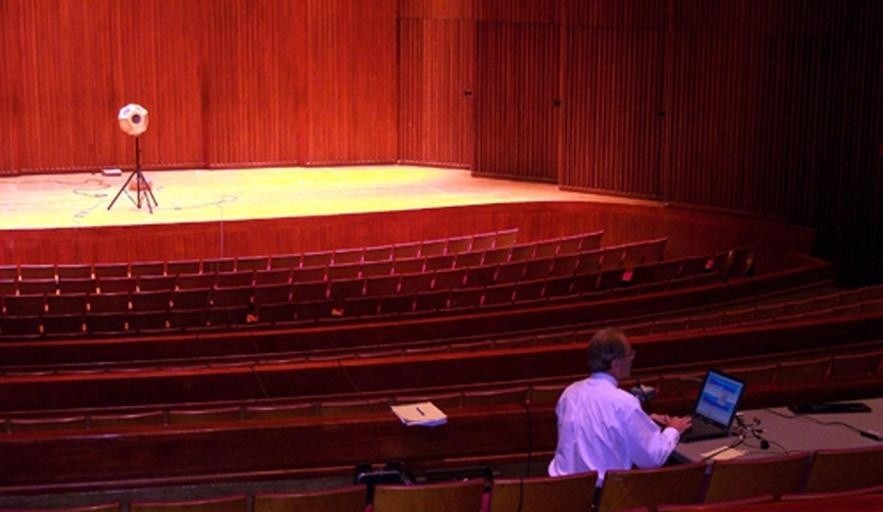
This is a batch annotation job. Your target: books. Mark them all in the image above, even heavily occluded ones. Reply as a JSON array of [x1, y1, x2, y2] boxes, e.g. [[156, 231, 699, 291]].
[[391, 402, 447, 427]]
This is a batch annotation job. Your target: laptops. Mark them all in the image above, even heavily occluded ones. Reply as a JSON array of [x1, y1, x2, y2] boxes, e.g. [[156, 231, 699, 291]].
[[655, 366, 747, 443]]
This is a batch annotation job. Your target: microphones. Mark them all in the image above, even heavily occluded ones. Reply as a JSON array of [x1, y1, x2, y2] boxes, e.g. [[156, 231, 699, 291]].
[[635, 383, 653, 415], [513, 395, 534, 478]]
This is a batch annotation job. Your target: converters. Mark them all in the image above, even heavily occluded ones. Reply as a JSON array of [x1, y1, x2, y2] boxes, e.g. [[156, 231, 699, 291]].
[[861, 430, 883, 440]]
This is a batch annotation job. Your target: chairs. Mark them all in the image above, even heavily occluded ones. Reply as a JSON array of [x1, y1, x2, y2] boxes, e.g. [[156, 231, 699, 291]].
[[0, 228, 882, 511]]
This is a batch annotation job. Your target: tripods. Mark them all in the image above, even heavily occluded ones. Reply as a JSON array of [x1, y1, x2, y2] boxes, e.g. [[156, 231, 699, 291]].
[[107, 137, 159, 211]]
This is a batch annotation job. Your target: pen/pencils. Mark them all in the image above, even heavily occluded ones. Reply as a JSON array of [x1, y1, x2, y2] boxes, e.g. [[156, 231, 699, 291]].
[[417, 407, 424, 416]]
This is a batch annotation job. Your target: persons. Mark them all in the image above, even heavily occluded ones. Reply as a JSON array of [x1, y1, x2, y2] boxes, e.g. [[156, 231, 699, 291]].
[[547, 327, 693, 512]]
